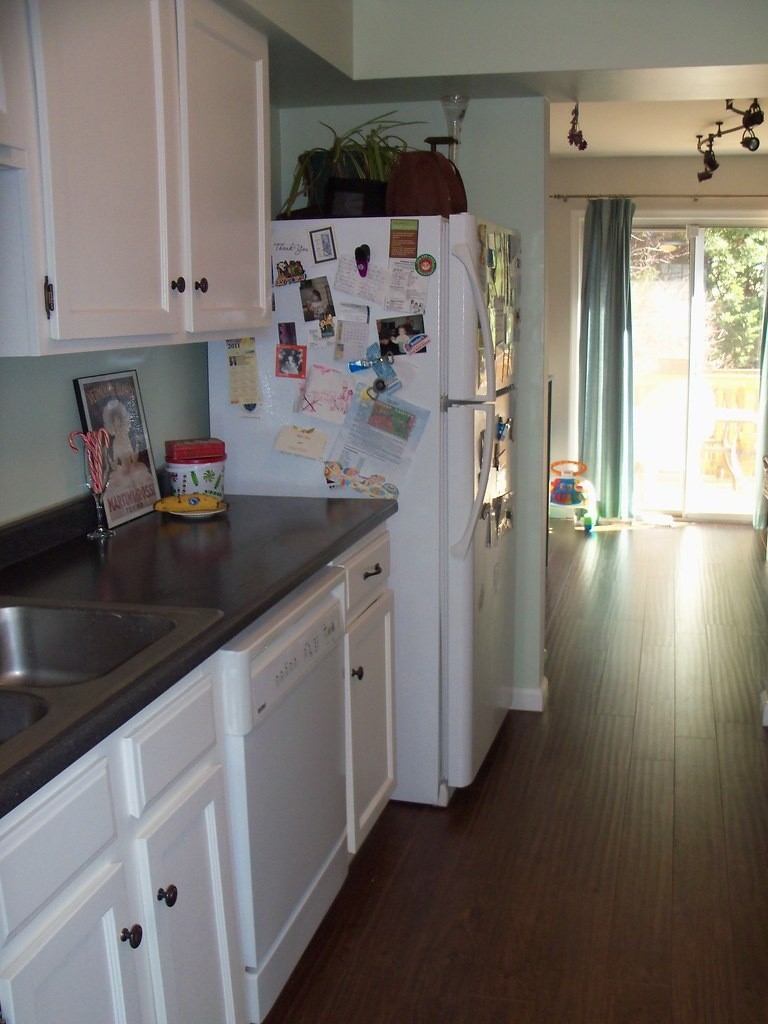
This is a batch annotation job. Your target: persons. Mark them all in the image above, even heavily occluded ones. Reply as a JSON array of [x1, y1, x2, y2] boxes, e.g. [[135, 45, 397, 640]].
[[278, 347, 303, 375], [306, 289, 326, 320], [376, 319, 413, 355]]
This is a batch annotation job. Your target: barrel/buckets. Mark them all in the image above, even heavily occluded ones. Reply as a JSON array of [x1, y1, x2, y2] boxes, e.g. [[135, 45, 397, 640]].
[[164, 453, 227, 501]]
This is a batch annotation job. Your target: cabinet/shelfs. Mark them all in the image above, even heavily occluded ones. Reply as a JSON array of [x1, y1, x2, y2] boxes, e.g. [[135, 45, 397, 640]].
[[0, 0, 271, 357], [0, 514, 397, 1024]]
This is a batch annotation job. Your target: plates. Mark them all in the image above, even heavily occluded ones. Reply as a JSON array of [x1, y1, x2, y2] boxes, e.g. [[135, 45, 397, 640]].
[[170, 509, 227, 518]]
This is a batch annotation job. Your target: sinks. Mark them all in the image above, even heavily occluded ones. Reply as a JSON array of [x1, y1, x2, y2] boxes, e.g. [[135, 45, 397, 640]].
[[0, 685, 53, 775], [0, 593, 224, 688]]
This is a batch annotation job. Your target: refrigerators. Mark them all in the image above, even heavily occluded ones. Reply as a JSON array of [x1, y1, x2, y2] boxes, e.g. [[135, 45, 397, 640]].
[[205, 213, 519, 806]]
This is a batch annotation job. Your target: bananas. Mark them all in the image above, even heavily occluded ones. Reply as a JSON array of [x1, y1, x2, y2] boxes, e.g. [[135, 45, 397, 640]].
[[153, 494, 228, 511]]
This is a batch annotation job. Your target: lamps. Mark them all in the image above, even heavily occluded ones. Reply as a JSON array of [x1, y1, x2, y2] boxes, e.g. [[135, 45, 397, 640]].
[[698, 134, 719, 182], [740, 98, 764, 151]]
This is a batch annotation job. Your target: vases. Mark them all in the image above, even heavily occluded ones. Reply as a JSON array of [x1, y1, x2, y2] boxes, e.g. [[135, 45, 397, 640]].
[[441, 95, 471, 168]]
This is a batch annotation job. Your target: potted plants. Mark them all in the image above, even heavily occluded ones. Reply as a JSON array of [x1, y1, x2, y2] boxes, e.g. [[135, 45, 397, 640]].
[[279, 110, 427, 218]]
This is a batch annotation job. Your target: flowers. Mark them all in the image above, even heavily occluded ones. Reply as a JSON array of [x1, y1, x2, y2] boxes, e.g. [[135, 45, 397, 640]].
[[567, 103, 588, 150]]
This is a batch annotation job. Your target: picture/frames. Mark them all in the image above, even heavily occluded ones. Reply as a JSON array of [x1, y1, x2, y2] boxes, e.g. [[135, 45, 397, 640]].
[[73, 370, 164, 530]]
